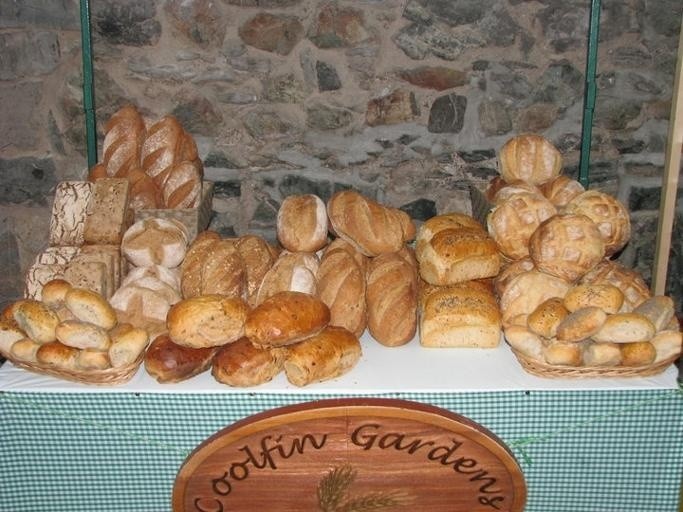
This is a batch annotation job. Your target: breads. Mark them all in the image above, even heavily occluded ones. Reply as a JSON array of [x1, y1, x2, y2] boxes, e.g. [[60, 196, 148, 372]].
[[0, 103, 683, 387]]
